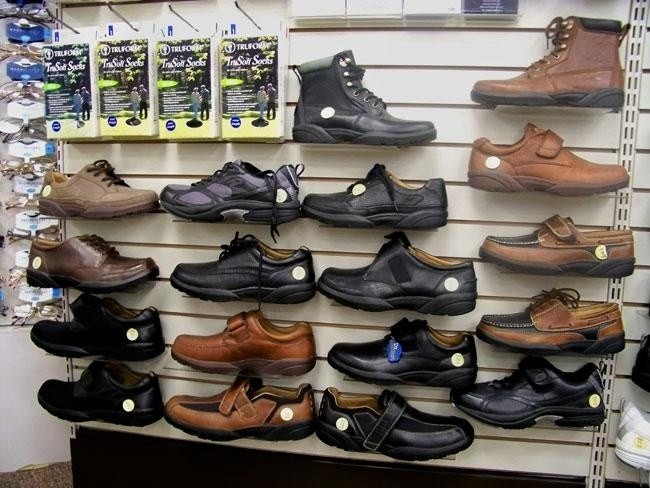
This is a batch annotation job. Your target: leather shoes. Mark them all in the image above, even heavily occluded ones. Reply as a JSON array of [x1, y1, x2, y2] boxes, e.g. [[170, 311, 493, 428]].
[[479, 216, 635, 282], [27, 233, 160, 287], [170, 309, 316, 377], [32, 293, 164, 362], [326, 318, 477, 387], [317, 388, 475, 459], [478, 288, 626, 357], [472, 120, 628, 198], [37, 362, 162, 427], [163, 371, 317, 443]]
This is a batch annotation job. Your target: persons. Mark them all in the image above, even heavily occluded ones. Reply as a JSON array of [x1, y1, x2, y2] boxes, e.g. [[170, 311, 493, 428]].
[[257, 86, 267, 118], [73, 89, 83, 120], [191, 87, 201, 118], [200, 84, 210, 120], [130, 87, 141, 117], [81, 86, 91, 120], [138, 84, 148, 119], [267, 83, 277, 120]]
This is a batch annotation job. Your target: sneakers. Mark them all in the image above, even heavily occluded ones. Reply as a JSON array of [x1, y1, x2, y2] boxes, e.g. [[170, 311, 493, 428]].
[[318, 231, 477, 315], [303, 163, 448, 230], [447, 355, 606, 429], [37, 159, 159, 218], [169, 231, 317, 310], [160, 159, 305, 242], [615, 396, 650, 470]]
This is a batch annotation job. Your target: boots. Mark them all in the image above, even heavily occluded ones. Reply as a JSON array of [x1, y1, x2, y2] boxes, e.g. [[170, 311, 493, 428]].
[[471, 15, 631, 108], [289, 49, 436, 146]]
